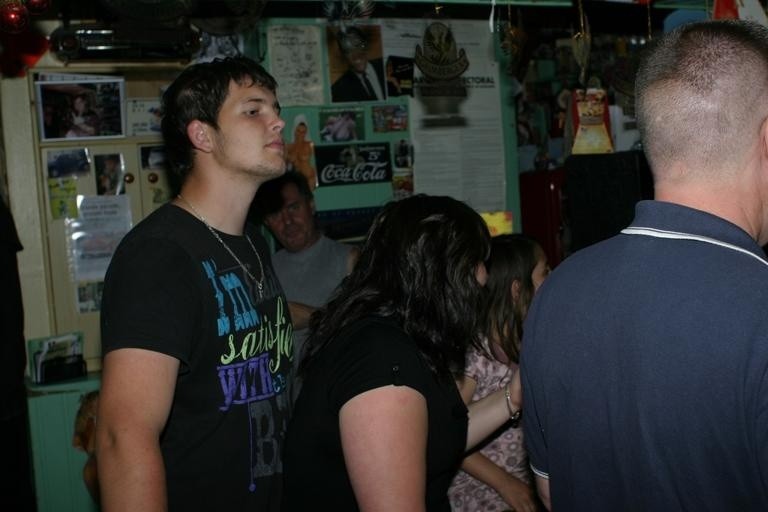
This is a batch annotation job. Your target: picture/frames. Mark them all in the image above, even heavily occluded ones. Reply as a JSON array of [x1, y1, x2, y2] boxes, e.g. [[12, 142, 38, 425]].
[[34, 78, 126, 142]]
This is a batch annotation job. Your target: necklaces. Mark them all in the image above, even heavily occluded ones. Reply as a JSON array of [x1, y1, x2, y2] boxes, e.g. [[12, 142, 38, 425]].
[[177, 192, 265, 307]]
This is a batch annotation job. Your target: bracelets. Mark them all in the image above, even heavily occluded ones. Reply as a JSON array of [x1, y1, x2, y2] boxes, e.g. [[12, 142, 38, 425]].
[[504, 384, 521, 420]]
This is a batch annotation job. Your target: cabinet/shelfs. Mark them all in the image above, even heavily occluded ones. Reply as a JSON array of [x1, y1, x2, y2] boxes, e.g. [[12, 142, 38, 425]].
[[25, 371, 101, 512], [519, 151, 654, 270]]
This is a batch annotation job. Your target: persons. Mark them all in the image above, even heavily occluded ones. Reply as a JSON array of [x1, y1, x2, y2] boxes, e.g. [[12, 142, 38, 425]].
[[520, 22, 768, 512], [331, 28, 385, 101], [278, 192, 520, 512], [395, 138, 413, 168], [260, 172, 357, 390], [447, 232, 555, 512], [97, 155, 125, 197], [93, 58, 295, 512], [321, 109, 360, 143], [64, 93, 100, 138], [283, 118, 316, 190]]
